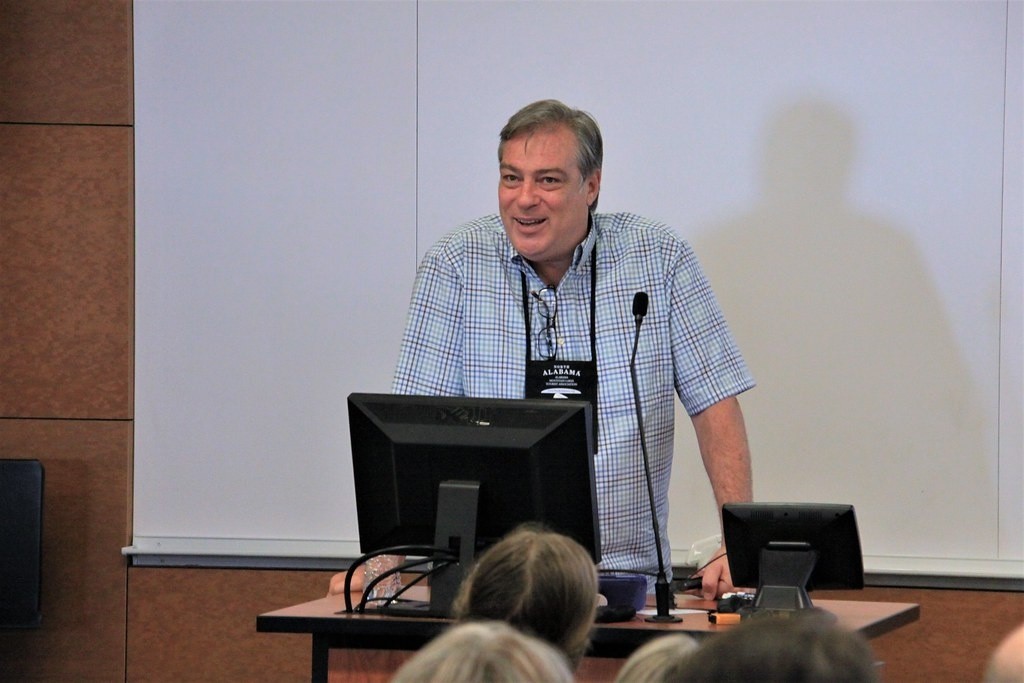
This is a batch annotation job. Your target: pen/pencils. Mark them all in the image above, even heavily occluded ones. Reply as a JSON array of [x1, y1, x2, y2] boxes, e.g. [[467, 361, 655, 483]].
[[709, 613, 790, 624]]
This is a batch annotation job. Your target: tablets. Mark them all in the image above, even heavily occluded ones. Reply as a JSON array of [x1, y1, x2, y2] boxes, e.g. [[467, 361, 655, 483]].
[[722, 502, 865, 591]]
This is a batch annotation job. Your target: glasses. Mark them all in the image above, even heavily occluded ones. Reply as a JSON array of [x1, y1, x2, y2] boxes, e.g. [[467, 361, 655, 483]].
[[530, 284, 558, 360]]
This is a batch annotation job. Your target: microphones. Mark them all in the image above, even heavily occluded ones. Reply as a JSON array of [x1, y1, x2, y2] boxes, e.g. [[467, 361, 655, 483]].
[[630, 292, 683, 624]]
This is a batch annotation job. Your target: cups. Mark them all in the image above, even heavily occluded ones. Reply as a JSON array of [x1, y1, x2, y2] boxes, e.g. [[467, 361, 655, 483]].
[[365, 555, 401, 606]]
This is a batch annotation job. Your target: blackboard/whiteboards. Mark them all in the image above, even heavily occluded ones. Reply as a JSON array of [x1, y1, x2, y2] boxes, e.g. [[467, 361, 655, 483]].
[[122, 0, 1023, 593]]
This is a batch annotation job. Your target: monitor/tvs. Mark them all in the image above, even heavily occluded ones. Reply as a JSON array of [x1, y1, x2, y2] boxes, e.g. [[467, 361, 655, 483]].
[[348, 393, 603, 620]]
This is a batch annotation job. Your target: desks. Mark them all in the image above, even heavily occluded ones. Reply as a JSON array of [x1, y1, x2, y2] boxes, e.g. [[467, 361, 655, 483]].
[[255, 586, 920, 683]]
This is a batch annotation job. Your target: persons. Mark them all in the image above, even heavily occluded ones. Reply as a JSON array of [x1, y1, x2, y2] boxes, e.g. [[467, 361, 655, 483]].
[[981, 625, 1024, 683], [323, 99, 755, 601], [388, 520, 880, 683]]
